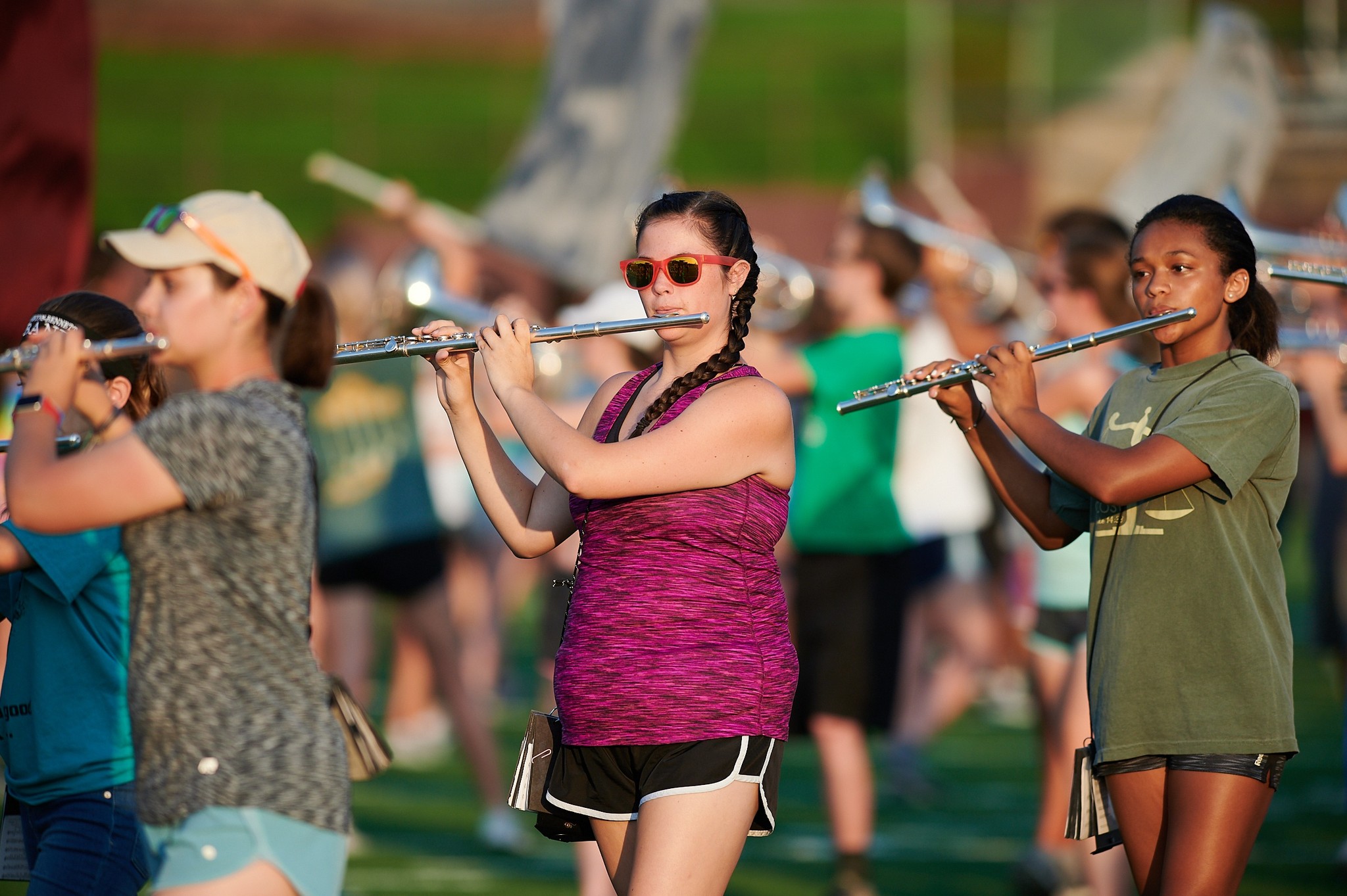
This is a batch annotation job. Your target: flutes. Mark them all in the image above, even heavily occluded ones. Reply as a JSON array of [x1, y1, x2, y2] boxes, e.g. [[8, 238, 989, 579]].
[[836, 306, 1197, 416], [1268, 259, 1347, 287], [329, 312, 710, 367], [0, 332, 170, 371]]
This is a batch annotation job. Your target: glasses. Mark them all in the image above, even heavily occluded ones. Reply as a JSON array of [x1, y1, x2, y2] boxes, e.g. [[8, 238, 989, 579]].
[[140, 205, 252, 289], [619, 253, 738, 290]]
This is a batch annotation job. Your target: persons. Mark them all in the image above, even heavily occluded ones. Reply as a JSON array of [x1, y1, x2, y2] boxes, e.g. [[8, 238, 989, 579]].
[[309, 196, 1347, 895], [412, 188, 797, 896], [3, 188, 356, 895], [0, 293, 170, 896], [904, 191, 1300, 896]]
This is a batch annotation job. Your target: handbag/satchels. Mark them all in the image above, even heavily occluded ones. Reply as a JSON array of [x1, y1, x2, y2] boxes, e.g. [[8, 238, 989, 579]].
[[327, 678, 393, 783]]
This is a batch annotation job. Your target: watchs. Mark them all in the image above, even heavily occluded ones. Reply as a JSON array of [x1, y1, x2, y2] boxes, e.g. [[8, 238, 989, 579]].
[[11, 391, 62, 425]]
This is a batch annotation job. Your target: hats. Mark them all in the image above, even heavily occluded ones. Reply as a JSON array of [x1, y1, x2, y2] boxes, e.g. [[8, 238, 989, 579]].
[[98, 188, 312, 309]]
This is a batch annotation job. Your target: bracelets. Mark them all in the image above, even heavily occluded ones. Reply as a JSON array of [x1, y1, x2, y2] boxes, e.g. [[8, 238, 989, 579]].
[[950, 401, 987, 433]]
